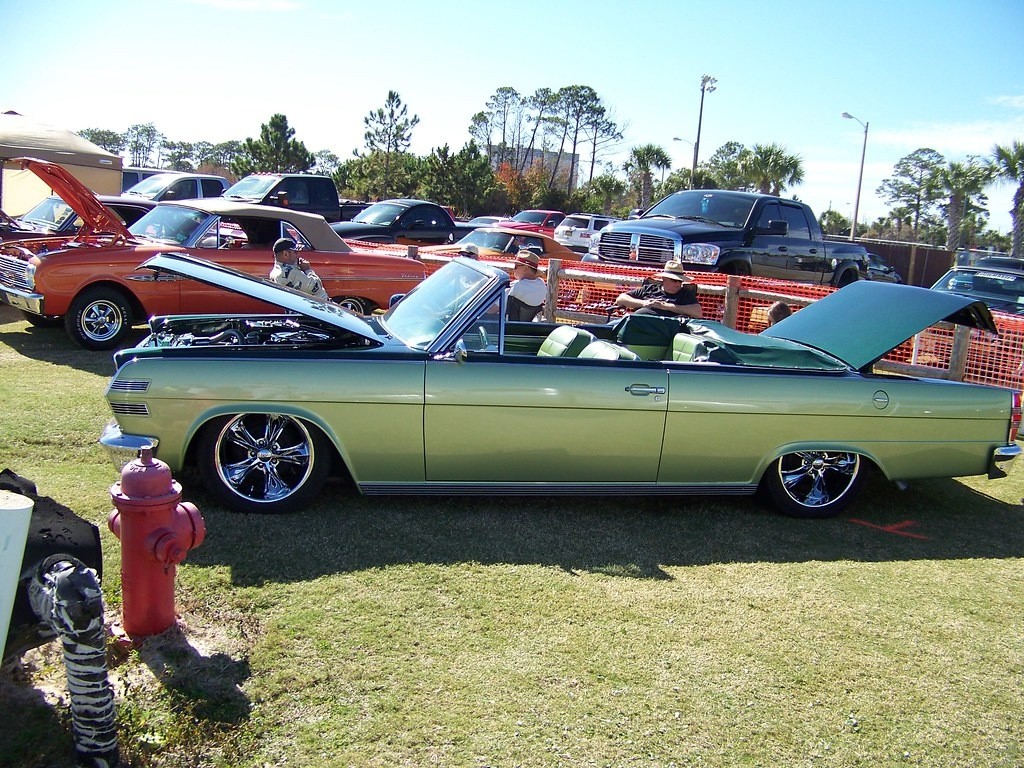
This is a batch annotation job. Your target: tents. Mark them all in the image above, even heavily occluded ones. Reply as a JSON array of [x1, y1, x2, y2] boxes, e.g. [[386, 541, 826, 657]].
[[0, 109, 122, 225]]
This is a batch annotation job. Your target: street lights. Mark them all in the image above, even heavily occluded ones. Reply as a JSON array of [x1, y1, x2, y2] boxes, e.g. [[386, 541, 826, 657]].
[[690, 74, 719, 188], [672, 137, 698, 193], [842, 112, 868, 241]]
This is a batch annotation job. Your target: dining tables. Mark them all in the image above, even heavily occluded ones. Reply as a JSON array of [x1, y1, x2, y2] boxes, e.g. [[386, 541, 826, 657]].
[[576, 340, 641, 361]]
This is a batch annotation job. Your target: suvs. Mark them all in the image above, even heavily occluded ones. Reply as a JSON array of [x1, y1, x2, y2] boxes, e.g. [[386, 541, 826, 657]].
[[553, 213, 623, 255]]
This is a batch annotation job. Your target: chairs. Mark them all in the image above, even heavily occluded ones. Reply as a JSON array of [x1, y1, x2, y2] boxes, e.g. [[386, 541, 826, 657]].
[[476, 295, 545, 322], [605, 277, 698, 324], [536, 325, 591, 358]]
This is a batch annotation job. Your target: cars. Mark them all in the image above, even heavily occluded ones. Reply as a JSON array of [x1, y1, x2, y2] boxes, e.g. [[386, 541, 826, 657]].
[[469, 215, 511, 225], [493, 209, 567, 239], [122, 166, 178, 192], [975, 255, 1024, 272], [930, 266, 1024, 318], [0, 195, 159, 244], [98, 250, 1022, 522], [120, 173, 231, 202], [329, 198, 494, 244], [1, 157, 428, 352], [867, 253, 903, 285]]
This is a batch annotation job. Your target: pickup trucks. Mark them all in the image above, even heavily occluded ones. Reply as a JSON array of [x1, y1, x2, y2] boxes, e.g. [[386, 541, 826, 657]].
[[581, 189, 869, 290], [216, 172, 370, 223]]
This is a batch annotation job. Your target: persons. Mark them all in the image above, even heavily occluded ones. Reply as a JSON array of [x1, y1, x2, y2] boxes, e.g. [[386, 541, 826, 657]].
[[458, 243, 479, 259], [269, 238, 329, 301], [768, 301, 791, 324], [606, 260, 702, 326], [486, 249, 546, 315]]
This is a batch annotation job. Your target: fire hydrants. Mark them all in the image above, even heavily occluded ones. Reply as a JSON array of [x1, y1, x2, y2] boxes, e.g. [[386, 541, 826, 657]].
[[104, 443, 206, 648]]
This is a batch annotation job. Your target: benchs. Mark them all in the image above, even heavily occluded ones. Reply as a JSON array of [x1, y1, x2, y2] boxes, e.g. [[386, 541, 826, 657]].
[[661, 333, 709, 362]]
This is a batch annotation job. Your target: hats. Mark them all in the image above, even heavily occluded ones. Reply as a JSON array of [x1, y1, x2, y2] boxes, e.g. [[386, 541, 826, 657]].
[[506, 249, 545, 276], [457, 243, 479, 255], [653, 260, 694, 281], [272, 239, 301, 254]]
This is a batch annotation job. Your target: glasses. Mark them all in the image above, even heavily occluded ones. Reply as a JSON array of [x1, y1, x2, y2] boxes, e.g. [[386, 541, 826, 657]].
[[662, 277, 683, 283], [461, 252, 476, 259], [514, 265, 524, 269]]
